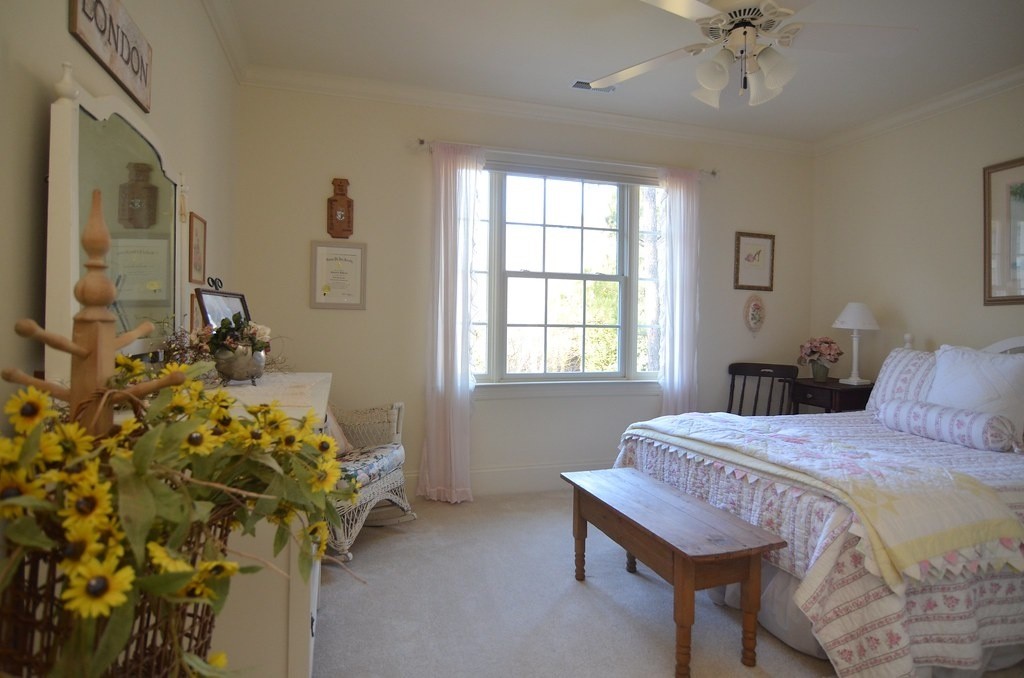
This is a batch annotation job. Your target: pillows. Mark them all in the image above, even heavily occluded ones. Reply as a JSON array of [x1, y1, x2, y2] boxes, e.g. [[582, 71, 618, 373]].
[[878, 399, 1024, 454], [324, 405, 354, 459], [865, 346, 937, 420], [931, 344, 1024, 443]]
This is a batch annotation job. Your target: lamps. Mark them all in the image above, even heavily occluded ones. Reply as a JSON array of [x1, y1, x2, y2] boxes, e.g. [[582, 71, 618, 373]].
[[690, 27, 800, 109], [831, 303, 880, 386]]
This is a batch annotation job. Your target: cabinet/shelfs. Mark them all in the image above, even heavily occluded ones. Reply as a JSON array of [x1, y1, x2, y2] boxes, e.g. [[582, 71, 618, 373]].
[[23, 371, 334, 677]]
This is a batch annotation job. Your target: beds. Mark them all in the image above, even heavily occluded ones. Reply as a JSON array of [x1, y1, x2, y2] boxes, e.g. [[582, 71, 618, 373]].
[[611, 337, 1024, 678]]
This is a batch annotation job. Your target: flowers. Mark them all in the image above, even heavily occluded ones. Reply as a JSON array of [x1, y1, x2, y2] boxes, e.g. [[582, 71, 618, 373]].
[[138, 312, 294, 389], [797, 336, 844, 370], [0, 352, 361, 678]]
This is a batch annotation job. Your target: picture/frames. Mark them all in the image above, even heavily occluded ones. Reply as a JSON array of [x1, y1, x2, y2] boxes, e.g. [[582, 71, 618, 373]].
[[190, 293, 205, 333], [734, 231, 775, 291], [188, 211, 207, 284], [194, 288, 251, 333], [982, 156, 1024, 306]]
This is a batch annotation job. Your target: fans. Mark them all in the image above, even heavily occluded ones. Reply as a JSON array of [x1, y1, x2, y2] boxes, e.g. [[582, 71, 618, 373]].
[[589, 0, 920, 96]]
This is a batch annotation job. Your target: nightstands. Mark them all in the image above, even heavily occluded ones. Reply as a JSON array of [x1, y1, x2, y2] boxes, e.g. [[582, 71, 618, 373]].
[[778, 377, 874, 415]]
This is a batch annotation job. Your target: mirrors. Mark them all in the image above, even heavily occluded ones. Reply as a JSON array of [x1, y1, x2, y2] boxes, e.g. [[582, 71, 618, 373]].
[[43, 61, 181, 410]]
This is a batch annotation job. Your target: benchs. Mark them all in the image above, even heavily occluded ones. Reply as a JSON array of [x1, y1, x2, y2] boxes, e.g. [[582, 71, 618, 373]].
[[558, 467, 789, 678]]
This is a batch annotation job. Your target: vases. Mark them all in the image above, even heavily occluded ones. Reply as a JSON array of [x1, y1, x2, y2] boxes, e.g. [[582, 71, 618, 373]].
[[213, 343, 266, 387], [811, 360, 829, 382]]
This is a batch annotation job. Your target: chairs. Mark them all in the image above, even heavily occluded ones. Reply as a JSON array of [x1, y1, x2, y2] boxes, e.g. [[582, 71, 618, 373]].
[[727, 363, 798, 416], [318, 399, 416, 564]]
